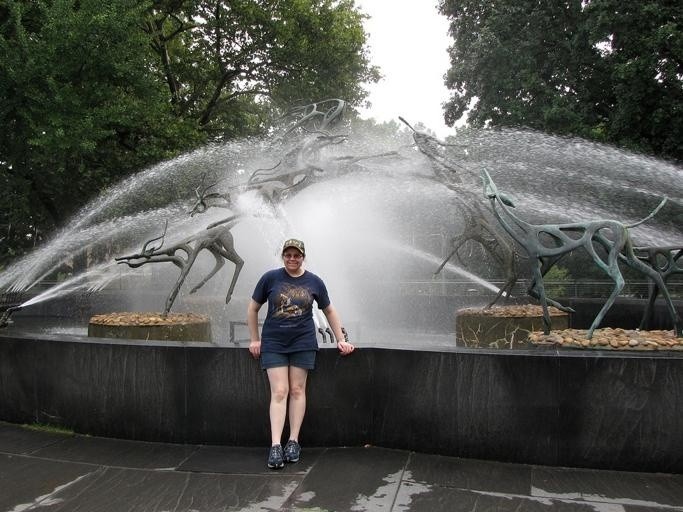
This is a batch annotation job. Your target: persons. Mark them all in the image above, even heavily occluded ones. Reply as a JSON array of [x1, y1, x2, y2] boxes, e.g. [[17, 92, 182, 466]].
[[246, 239, 355, 470]]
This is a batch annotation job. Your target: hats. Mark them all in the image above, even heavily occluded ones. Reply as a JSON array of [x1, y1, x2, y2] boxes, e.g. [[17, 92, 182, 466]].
[[282, 239, 305, 254]]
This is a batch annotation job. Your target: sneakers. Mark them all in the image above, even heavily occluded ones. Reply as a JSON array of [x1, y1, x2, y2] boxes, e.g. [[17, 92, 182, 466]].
[[267, 444, 284, 468], [283, 440, 302, 462]]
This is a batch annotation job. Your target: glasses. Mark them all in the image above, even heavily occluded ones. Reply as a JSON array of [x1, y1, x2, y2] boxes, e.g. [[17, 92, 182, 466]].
[[283, 251, 303, 259]]
